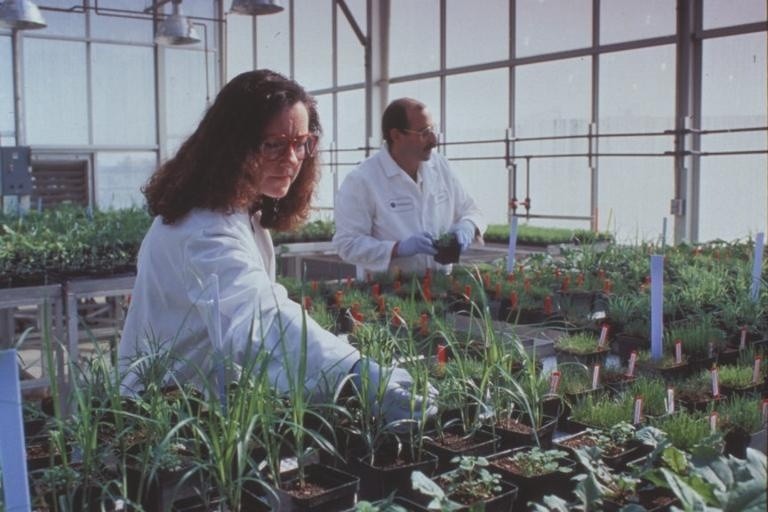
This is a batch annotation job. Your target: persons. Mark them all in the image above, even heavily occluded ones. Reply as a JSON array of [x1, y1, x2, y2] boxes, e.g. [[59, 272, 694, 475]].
[[106, 69, 439, 424], [325, 92, 489, 282]]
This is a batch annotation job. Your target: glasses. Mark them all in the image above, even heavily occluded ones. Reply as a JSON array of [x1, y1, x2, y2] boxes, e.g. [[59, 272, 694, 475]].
[[401, 123, 439, 144], [256, 134, 320, 164]]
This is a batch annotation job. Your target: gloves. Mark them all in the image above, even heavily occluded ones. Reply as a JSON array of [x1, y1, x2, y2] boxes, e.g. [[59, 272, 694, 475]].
[[396, 227, 442, 257], [446, 216, 478, 254], [349, 354, 440, 438]]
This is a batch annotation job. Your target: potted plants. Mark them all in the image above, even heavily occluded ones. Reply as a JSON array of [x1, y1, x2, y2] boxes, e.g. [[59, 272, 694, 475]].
[[2, 200, 766, 512]]
